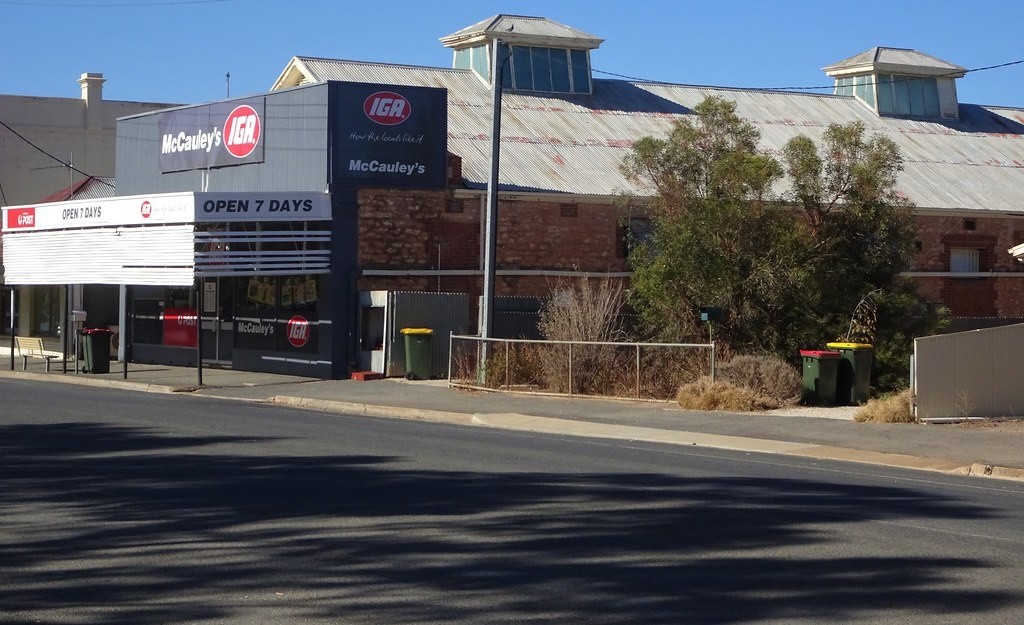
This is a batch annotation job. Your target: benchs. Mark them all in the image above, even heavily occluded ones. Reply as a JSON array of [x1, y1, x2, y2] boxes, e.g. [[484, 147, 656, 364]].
[[14, 336, 63, 373]]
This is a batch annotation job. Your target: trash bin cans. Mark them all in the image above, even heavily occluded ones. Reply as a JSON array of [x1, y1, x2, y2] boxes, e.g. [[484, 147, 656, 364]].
[[800, 349, 841, 407], [77, 328, 115, 374], [825, 342, 873, 405], [400, 327, 434, 380]]
